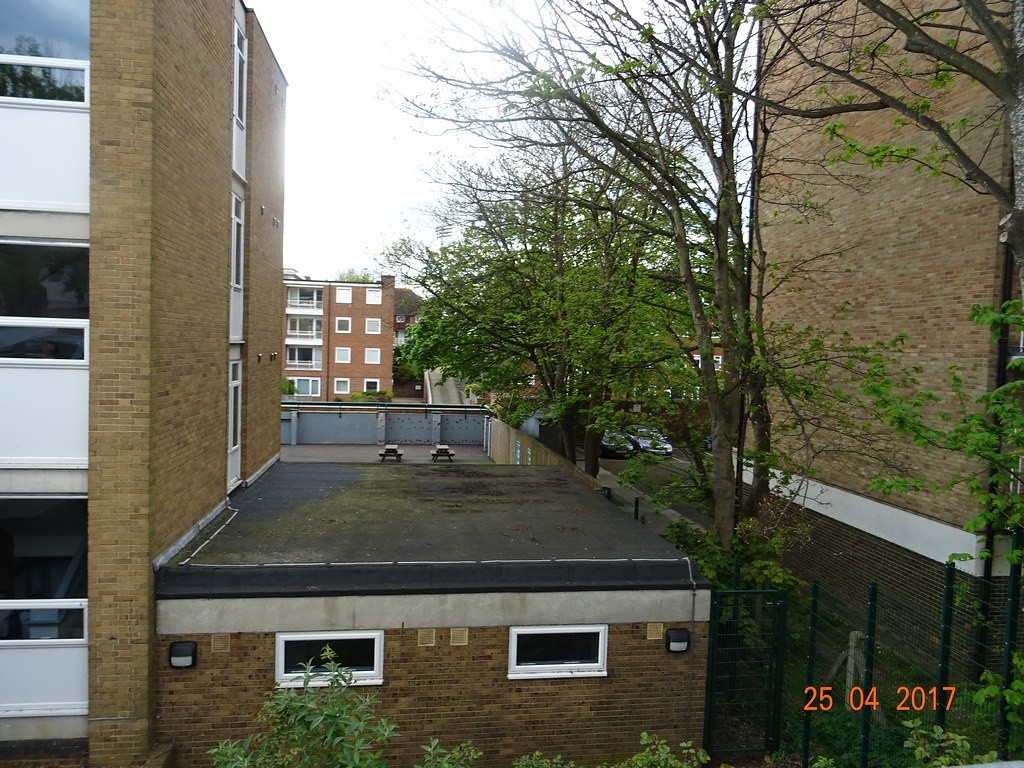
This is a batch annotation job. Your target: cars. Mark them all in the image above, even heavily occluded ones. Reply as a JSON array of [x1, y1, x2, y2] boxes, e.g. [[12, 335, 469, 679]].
[[599, 429, 634, 460], [623, 425, 674, 456]]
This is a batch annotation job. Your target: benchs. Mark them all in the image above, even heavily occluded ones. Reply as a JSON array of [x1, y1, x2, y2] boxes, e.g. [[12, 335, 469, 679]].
[[378, 450, 403, 462], [430, 450, 455, 462]]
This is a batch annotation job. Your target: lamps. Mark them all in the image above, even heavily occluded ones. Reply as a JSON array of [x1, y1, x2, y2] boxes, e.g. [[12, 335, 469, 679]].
[[666, 628, 689, 653], [169, 641, 197, 669]]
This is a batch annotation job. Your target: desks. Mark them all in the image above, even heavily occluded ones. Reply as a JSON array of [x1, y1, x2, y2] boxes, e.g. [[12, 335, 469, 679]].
[[431, 445, 454, 462], [381, 445, 401, 462]]
